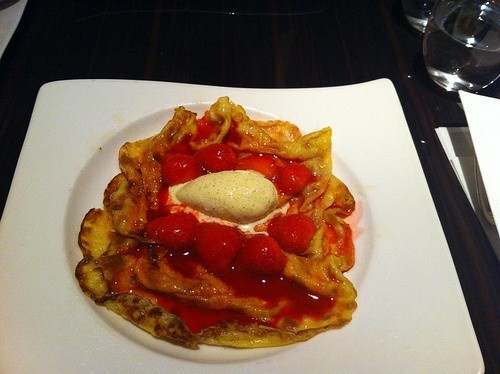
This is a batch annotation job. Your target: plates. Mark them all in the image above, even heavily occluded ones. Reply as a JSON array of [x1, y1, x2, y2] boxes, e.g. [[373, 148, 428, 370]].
[[458, 83, 500, 232], [0, 78, 486, 373]]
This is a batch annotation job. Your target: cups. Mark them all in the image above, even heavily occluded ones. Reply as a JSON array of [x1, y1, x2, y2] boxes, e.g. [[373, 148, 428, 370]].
[[403, 0, 500, 92]]
